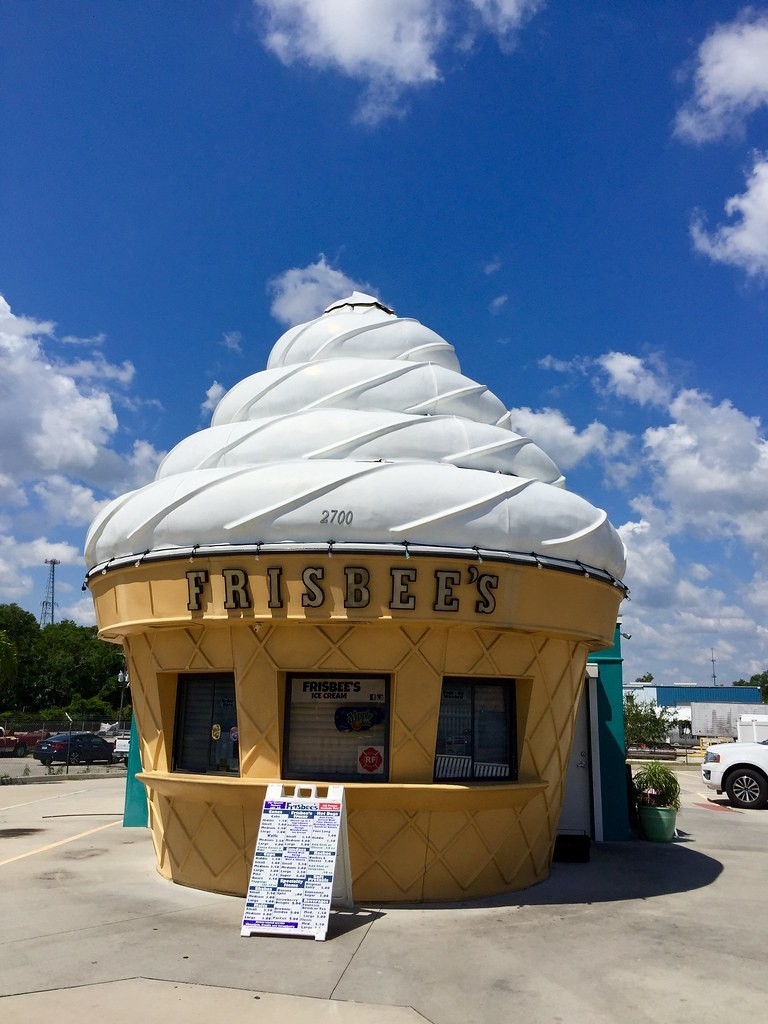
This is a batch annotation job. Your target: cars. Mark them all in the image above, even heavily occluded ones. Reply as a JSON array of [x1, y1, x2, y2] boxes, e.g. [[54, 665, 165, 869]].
[[0, 726, 27, 758], [32, 732, 120, 765], [98, 726, 120, 736], [700, 737, 768, 808]]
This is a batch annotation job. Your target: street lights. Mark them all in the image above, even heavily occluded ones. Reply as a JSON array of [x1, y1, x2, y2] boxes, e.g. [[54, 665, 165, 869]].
[[115, 670, 130, 732]]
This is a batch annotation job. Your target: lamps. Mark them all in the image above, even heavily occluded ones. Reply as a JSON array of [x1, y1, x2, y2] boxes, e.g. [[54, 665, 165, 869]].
[[471, 546, 484, 564], [574, 559, 590, 579], [134, 549, 150, 568], [530, 551, 543, 570], [618, 580, 631, 594], [102, 557, 115, 576], [603, 569, 618, 587], [82, 581, 88, 594], [255, 541, 265, 562], [401, 540, 411, 560], [189, 544, 200, 563], [327, 540, 336, 558], [625, 595, 631, 601], [84, 565, 97, 582]]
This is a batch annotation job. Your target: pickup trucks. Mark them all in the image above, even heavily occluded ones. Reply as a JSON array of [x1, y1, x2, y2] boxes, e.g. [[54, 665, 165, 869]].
[[111, 737, 130, 768]]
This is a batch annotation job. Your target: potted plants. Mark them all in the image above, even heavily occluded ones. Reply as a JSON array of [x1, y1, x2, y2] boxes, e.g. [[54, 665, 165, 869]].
[[632, 760, 681, 841]]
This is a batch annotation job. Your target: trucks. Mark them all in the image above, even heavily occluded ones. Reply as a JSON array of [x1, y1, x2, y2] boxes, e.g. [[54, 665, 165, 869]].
[[5, 728, 50, 745]]
